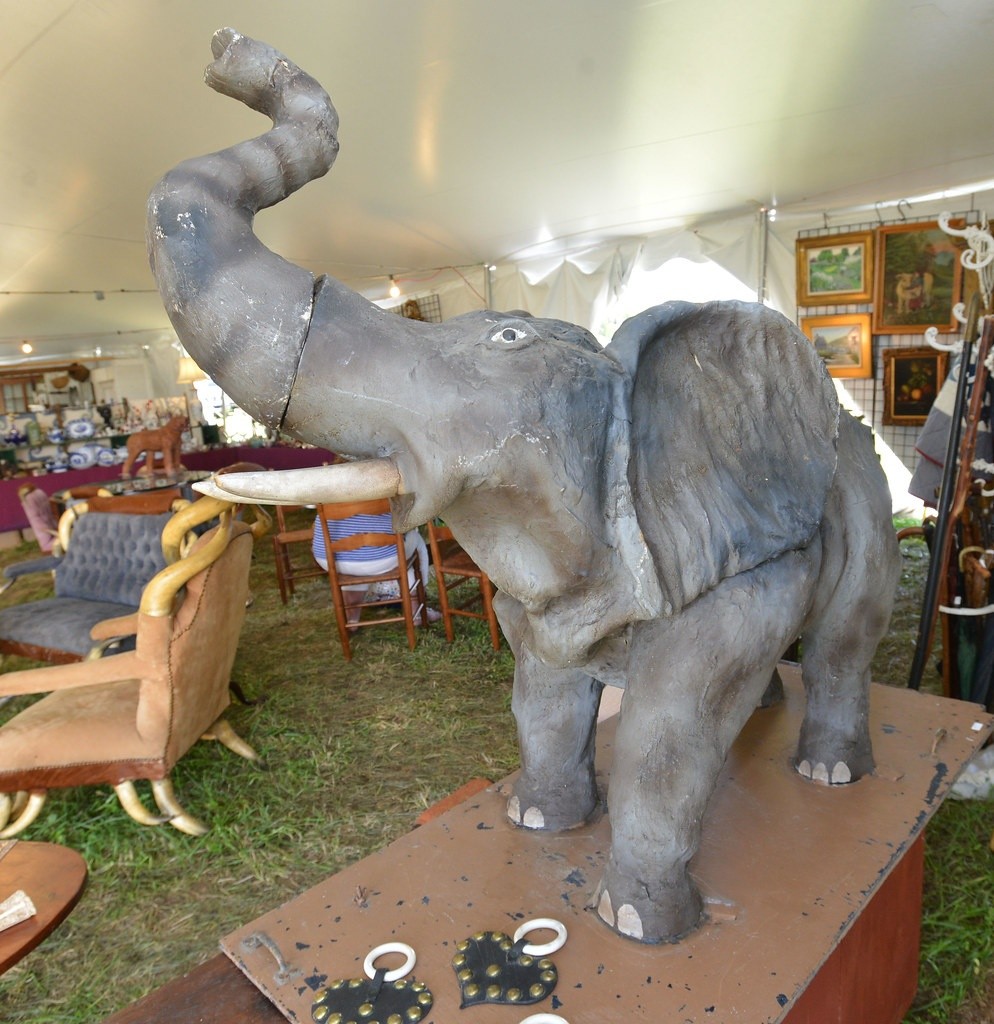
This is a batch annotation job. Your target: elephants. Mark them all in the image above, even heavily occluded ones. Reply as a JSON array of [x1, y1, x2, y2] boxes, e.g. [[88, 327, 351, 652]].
[[143, 30, 900, 946]]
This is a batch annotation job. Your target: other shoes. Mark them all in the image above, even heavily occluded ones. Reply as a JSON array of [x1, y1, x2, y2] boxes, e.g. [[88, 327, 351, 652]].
[[413, 607, 441, 627]]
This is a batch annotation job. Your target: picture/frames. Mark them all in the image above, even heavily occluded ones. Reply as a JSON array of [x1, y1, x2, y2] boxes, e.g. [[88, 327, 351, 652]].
[[800, 312, 872, 380], [870, 218, 966, 334], [883, 346, 948, 427], [795, 229, 875, 306]]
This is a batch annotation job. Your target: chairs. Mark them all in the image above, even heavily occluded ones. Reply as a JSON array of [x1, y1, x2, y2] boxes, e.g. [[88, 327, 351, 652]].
[[0, 498, 197, 706], [316, 497, 427, 661], [0, 496, 274, 839], [427, 518, 500, 654], [272, 500, 333, 605]]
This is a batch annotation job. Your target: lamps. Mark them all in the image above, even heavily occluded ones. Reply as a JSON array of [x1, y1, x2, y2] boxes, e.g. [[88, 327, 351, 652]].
[[174, 339, 208, 386]]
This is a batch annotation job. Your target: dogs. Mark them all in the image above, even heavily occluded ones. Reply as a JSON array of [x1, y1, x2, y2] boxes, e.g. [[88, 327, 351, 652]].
[[122, 416, 190, 479]]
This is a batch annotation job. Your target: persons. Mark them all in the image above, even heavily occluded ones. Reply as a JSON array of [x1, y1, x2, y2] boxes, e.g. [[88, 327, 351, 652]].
[[312, 458, 443, 631]]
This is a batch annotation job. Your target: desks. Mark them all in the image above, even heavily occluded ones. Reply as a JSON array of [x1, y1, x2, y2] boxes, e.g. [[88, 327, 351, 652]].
[[0, 840, 88, 978], [0, 444, 337, 534]]
